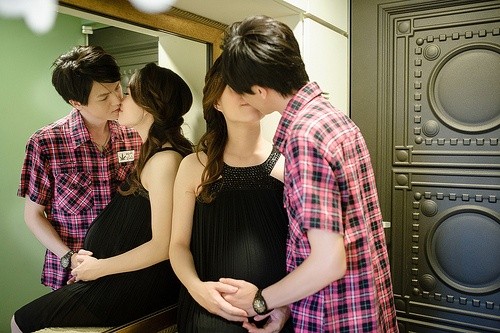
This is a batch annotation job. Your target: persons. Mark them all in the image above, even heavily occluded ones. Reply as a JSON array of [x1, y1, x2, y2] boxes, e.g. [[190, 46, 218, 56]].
[[168, 53, 295, 333], [218, 14, 399, 333], [17, 44, 144, 292], [10, 61, 199, 333]]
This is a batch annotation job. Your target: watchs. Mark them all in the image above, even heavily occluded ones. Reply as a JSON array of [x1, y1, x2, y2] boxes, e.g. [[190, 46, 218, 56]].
[[60, 249, 76, 273], [252, 289, 274, 315]]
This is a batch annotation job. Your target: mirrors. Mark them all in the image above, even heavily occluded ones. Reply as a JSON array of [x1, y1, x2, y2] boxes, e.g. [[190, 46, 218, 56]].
[[0, 0, 231, 333]]
